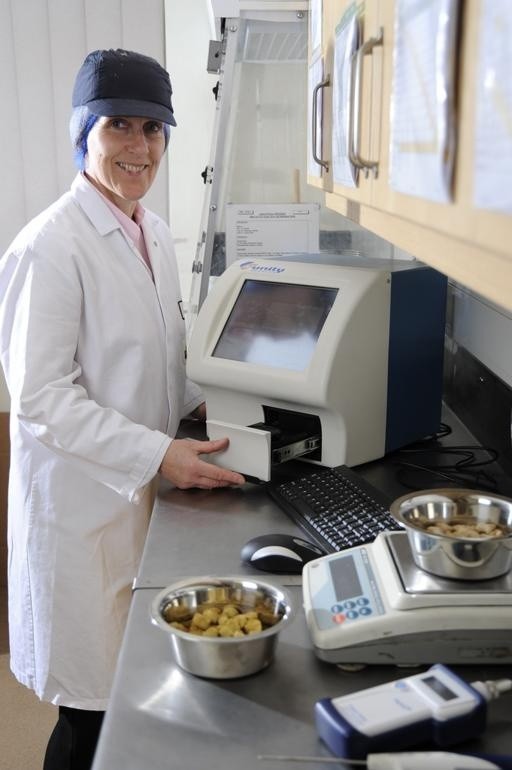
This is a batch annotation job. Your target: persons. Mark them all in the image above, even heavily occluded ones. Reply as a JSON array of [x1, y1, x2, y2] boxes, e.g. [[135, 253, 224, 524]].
[[0, 48, 254, 770]]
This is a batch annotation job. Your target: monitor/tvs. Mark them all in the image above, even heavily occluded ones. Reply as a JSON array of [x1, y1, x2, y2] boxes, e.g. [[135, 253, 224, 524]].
[[209, 278, 341, 377]]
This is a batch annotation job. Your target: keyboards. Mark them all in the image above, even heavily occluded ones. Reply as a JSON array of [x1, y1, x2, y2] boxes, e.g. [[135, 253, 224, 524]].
[[265, 464, 406, 556]]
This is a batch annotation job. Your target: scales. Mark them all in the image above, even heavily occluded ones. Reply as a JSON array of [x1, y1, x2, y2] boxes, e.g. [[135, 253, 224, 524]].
[[301, 531, 511, 671]]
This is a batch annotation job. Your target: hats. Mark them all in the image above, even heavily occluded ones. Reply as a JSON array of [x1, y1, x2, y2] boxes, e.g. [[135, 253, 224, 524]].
[[71, 48, 177, 128]]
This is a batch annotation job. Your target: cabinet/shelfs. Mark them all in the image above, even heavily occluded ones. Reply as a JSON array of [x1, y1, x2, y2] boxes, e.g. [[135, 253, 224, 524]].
[[306, 0, 512, 310]]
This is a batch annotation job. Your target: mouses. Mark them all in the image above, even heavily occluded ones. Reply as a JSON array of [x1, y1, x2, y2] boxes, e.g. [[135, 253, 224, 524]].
[[240, 534, 329, 575]]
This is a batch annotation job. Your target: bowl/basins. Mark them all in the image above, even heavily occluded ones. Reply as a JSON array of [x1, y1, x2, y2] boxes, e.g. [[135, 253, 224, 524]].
[[392, 489, 512, 580], [148, 577, 292, 680]]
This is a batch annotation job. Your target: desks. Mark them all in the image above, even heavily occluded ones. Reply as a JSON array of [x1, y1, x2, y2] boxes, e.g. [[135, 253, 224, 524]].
[[90, 401, 512, 770]]
